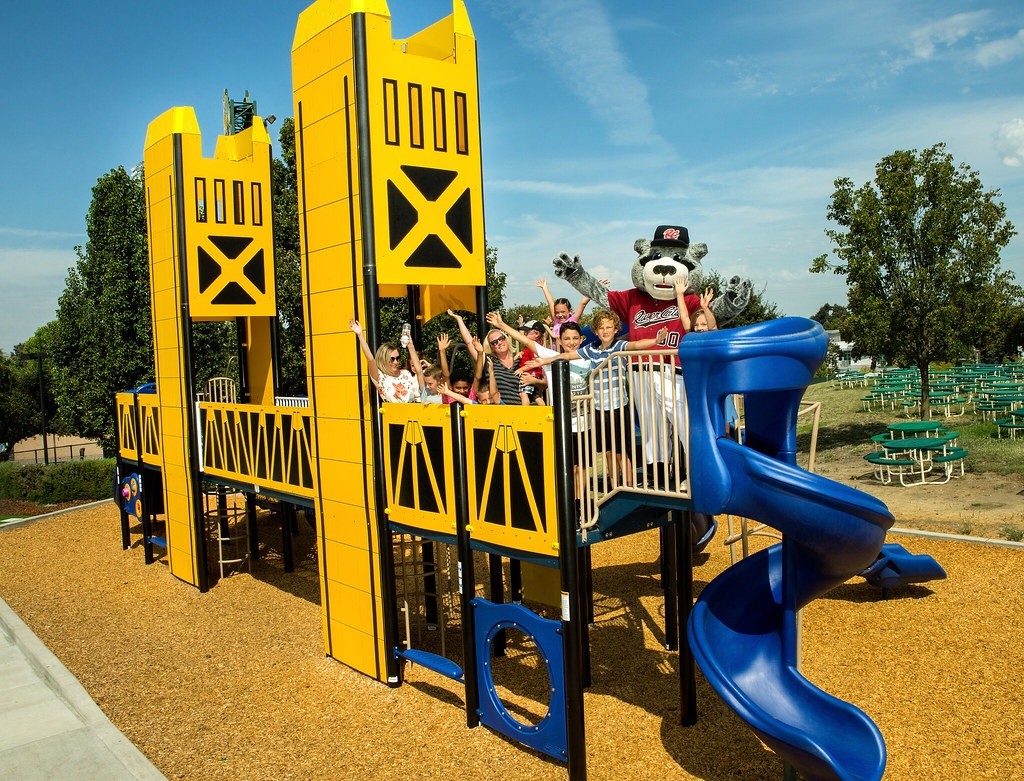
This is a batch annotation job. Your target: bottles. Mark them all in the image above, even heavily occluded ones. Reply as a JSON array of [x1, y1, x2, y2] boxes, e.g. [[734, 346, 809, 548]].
[[402, 324, 410, 348]]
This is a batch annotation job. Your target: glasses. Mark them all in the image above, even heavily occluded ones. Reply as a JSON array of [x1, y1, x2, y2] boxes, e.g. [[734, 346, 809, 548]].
[[524, 331, 535, 336], [389, 355, 401, 363], [490, 335, 506, 346]]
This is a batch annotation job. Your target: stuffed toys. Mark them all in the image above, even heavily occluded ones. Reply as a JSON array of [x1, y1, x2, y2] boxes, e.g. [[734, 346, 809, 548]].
[[553, 225, 752, 488]]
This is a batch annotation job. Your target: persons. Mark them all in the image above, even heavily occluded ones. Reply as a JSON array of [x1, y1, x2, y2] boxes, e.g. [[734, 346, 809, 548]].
[[486, 312, 591, 528], [536, 278, 610, 352], [349, 309, 548, 406], [675, 278, 739, 423], [524, 311, 668, 489]]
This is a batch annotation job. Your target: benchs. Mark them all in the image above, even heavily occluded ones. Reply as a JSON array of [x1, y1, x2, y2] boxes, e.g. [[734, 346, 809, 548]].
[[836, 362, 1024, 487]]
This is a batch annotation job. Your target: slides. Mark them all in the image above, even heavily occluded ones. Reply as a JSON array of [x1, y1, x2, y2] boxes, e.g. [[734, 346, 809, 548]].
[[691, 436, 948, 780]]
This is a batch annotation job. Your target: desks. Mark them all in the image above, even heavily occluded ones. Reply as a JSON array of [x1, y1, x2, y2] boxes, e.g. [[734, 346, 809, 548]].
[[977, 378, 1003, 384], [948, 367, 976, 372], [986, 383, 1024, 392], [881, 437, 946, 483], [947, 375, 979, 383], [878, 374, 907, 377], [928, 371, 952, 381], [887, 421, 942, 462], [913, 393, 953, 418], [1002, 365, 1022, 372], [881, 381, 912, 389], [929, 383, 955, 392], [985, 391, 1024, 402], [991, 396, 1024, 411], [871, 390, 902, 398]]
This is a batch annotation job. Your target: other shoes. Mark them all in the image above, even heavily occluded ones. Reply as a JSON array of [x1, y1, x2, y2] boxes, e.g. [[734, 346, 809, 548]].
[[575, 497, 593, 532], [680, 479, 687, 491]]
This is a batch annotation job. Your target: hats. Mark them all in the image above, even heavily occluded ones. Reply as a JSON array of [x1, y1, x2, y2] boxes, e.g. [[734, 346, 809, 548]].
[[516, 320, 546, 334]]
